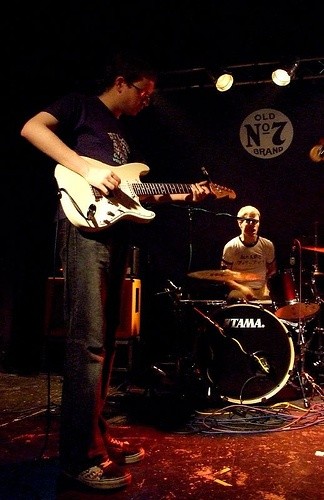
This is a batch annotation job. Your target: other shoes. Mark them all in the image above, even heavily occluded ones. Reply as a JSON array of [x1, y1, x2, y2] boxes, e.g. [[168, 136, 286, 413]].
[[104, 437, 145, 463], [74, 458, 130, 488]]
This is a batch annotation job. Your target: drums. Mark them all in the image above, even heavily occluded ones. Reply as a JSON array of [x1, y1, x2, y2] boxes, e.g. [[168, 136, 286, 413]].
[[196, 303, 296, 406], [266, 270, 320, 319]]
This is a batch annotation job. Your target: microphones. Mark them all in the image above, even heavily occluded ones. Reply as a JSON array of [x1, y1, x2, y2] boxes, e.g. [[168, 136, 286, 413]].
[[256, 357, 270, 373], [290, 240, 296, 265], [250, 220, 258, 226]]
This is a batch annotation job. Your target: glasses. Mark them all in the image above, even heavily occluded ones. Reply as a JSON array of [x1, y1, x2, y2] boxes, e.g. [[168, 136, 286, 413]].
[[128, 83, 153, 101], [243, 217, 260, 224]]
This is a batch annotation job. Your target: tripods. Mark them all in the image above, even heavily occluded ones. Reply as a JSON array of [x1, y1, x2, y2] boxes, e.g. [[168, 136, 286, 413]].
[[285, 319, 324, 407]]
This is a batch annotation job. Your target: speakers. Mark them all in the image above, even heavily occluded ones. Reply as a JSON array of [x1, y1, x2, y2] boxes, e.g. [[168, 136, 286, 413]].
[[46, 276, 142, 338]]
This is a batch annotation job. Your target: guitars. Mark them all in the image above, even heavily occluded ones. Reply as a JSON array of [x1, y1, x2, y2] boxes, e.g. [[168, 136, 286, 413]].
[[54, 155, 237, 231]]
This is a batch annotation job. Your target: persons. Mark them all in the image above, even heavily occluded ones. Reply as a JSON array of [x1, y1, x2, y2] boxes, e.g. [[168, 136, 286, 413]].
[[20, 55, 210, 488], [222, 206, 277, 307]]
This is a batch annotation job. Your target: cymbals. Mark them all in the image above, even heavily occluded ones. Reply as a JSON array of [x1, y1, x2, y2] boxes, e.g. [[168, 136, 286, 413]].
[[186, 269, 265, 282], [301, 246, 324, 253]]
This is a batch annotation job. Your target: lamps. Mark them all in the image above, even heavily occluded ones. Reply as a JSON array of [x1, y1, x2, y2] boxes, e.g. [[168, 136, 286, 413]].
[[208, 64, 234, 92], [271, 54, 303, 86]]
